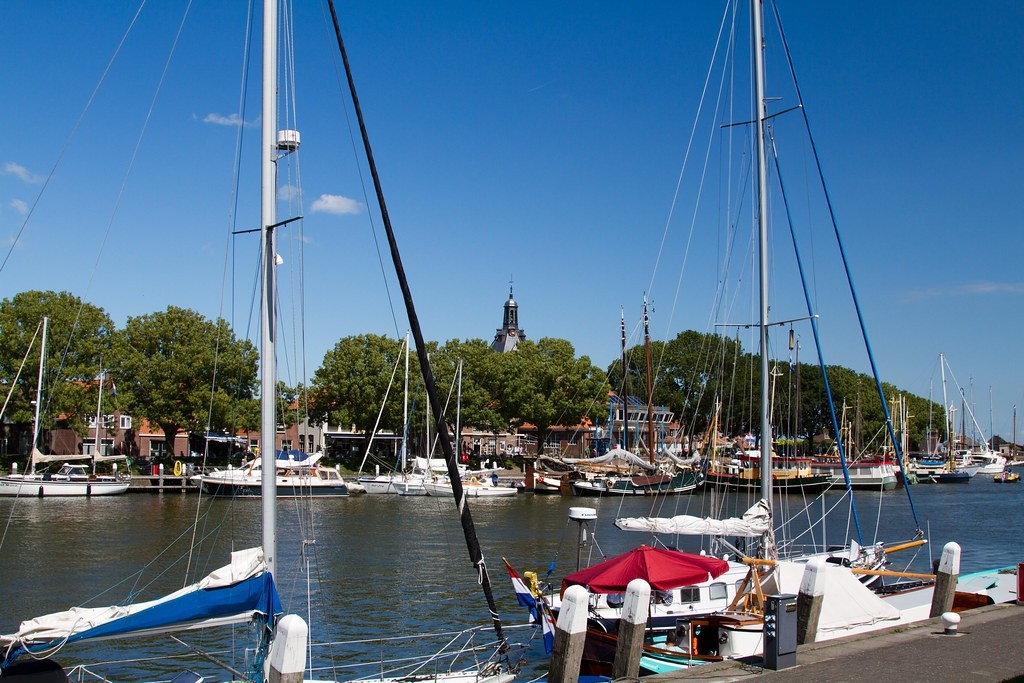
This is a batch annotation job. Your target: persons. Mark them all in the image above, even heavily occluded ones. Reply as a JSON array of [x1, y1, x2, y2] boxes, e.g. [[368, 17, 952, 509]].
[[180, 451, 184, 456], [490, 472, 498, 486], [654, 632, 686, 653]]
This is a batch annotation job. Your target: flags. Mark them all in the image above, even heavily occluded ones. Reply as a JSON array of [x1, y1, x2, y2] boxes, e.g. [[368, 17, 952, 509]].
[[528, 598, 555, 654], [504, 563, 535, 608]]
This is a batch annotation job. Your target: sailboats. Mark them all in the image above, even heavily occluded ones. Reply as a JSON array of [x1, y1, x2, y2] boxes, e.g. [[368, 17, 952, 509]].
[[526, 1, 1023, 674], [0, 0, 556, 683], [0, 286, 1010, 500]]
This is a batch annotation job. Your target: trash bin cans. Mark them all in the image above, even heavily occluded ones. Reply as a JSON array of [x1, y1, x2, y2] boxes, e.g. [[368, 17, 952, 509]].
[[1017, 562, 1024, 604]]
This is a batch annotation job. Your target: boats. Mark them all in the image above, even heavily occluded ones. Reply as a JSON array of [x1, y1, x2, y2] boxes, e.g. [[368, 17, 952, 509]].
[[994, 472, 1019, 482]]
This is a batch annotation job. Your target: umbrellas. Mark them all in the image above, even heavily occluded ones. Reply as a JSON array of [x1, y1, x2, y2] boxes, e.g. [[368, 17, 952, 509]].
[[562, 544, 729, 644]]
